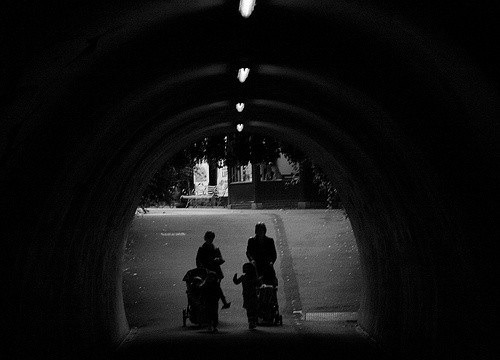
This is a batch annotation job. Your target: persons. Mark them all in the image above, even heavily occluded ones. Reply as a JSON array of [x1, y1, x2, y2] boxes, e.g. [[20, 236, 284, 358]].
[[203, 271, 226, 332], [246, 222, 277, 264], [233, 263, 262, 329], [196, 231, 225, 282]]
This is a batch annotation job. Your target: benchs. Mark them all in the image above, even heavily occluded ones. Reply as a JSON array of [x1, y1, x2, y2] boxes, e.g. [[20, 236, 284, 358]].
[[180, 185, 218, 209]]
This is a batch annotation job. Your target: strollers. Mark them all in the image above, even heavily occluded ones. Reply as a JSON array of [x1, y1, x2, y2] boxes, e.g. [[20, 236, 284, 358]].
[[253, 262, 283, 326], [182, 268, 212, 330]]
